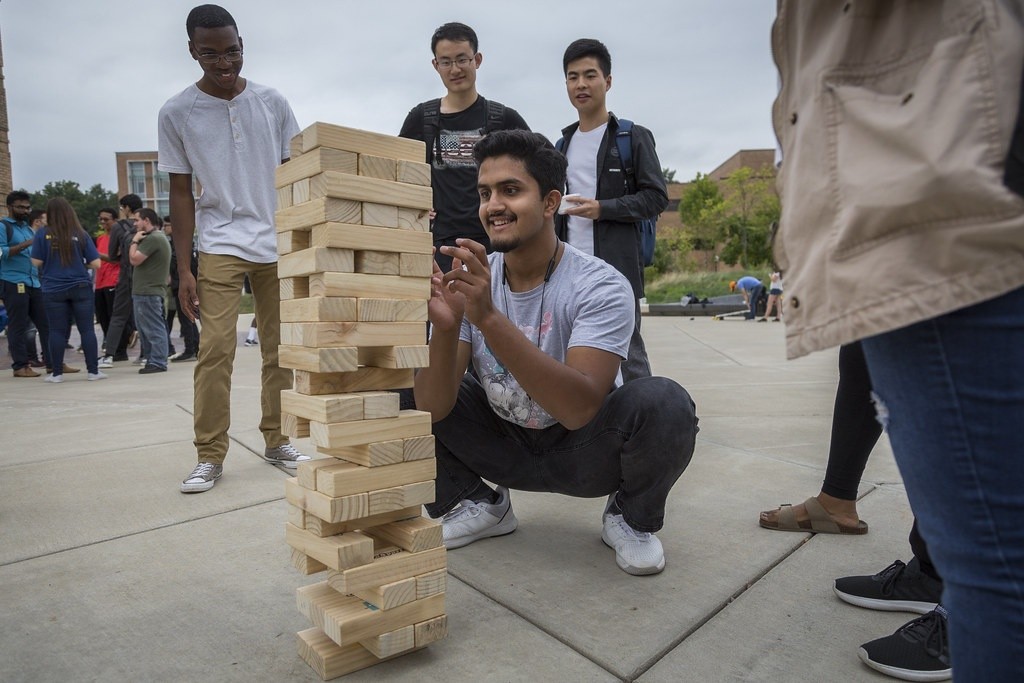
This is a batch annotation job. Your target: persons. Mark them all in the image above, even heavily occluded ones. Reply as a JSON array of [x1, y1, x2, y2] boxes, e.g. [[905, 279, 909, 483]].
[[397, 21, 532, 343], [728, 0, 1024, 683], [554, 39, 670, 392], [0, 191, 259, 382], [159, 4, 312, 493], [387, 131, 700, 575]]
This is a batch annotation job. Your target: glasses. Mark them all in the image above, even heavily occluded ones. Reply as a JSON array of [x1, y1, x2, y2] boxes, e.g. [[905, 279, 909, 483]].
[[99, 217, 114, 222], [191, 37, 243, 64], [164, 224, 171, 228], [435, 53, 476, 70], [12, 205, 32, 213]]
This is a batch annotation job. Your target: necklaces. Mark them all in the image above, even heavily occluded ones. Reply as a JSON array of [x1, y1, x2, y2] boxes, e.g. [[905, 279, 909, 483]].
[[501, 236, 560, 349]]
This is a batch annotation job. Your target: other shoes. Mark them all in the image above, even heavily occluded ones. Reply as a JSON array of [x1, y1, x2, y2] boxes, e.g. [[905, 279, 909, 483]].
[[47, 365, 80, 374], [28, 358, 43, 367], [170, 351, 197, 363], [132, 357, 149, 365], [101, 344, 106, 353], [76, 347, 84, 353], [66, 344, 74, 349], [757, 319, 768, 321], [773, 319, 780, 322]]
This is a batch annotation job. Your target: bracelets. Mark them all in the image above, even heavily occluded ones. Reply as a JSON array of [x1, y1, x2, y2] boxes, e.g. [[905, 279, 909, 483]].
[[18, 243, 25, 251], [128, 240, 140, 246]]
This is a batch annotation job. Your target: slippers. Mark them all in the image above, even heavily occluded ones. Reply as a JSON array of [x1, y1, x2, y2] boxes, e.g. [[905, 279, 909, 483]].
[[759, 496, 868, 535]]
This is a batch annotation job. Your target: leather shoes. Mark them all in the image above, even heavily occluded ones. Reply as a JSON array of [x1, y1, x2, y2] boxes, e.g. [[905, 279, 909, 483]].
[[139, 364, 163, 375]]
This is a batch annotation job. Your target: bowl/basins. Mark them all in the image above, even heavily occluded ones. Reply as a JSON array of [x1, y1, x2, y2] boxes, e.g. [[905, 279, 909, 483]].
[[557, 193, 581, 215]]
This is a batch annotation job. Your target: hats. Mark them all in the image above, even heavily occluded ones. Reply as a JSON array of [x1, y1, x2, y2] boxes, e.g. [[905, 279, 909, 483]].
[[730, 281, 736, 292]]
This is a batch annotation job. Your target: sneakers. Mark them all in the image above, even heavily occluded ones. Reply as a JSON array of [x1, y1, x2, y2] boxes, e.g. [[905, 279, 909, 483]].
[[440, 485, 518, 549], [245, 338, 259, 346], [13, 366, 41, 377], [834, 555, 944, 615], [97, 356, 113, 368], [265, 444, 312, 468], [88, 370, 108, 381], [44, 372, 65, 382], [601, 508, 665, 574], [181, 462, 223, 492], [129, 330, 139, 348], [859, 604, 954, 682]]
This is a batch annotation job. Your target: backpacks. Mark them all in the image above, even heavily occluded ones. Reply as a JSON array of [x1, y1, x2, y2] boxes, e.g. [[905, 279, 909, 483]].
[[118, 219, 138, 281], [555, 119, 657, 268]]
